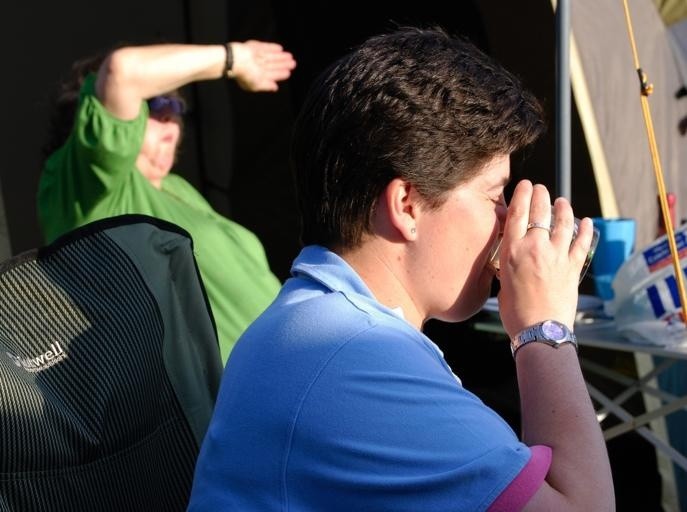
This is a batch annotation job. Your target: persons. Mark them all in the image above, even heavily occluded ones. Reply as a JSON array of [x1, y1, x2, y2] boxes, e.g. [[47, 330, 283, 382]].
[[33, 38, 299, 370], [187, 17, 618, 511]]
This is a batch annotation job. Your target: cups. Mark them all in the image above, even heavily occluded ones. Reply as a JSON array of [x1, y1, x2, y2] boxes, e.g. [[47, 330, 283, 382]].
[[488, 195, 601, 289], [587, 217, 636, 302]]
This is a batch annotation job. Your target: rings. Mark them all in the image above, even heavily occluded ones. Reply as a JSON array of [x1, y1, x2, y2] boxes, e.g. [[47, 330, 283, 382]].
[[526, 222, 550, 232]]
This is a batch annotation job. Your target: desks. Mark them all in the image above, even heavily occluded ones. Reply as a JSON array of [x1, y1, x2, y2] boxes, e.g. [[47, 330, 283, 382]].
[[471, 303, 685, 477]]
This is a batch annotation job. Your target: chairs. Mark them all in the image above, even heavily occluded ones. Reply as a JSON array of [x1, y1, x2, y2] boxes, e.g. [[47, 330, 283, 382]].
[[0, 207, 225, 509]]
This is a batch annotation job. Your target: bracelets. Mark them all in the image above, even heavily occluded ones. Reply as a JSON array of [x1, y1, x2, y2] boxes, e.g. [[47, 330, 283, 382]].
[[222, 41, 234, 81]]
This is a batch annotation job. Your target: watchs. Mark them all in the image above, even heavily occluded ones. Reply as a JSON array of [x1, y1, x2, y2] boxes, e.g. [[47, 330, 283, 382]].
[[509, 320, 581, 359]]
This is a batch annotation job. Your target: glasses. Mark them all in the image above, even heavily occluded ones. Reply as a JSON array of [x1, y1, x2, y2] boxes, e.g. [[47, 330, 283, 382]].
[[146, 95, 189, 116]]
